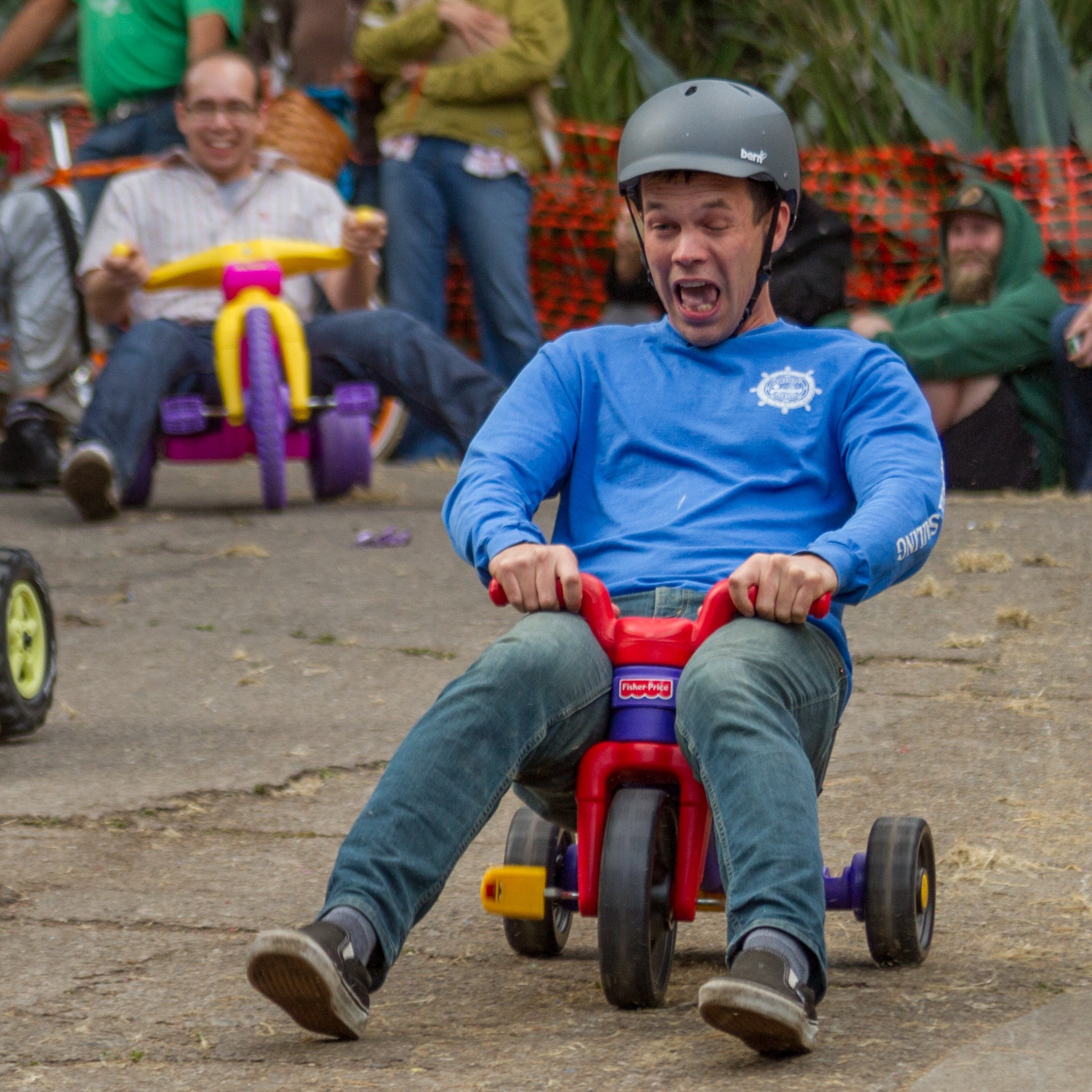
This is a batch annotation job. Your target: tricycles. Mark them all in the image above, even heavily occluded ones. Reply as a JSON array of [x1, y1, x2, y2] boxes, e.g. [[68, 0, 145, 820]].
[[479, 568, 939, 1013], [109, 206, 381, 514]]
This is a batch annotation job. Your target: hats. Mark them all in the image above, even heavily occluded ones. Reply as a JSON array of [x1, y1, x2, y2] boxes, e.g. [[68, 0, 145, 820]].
[[933, 185, 1002, 223]]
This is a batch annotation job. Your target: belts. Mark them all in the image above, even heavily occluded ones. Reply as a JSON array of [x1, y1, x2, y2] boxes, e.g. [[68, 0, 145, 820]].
[[100, 90, 183, 128]]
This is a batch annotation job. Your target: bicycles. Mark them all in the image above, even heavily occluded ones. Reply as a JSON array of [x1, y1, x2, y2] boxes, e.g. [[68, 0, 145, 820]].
[[0, 78, 408, 487]]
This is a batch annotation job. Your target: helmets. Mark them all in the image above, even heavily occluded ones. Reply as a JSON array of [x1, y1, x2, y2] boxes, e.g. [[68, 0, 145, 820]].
[[618, 78, 803, 229]]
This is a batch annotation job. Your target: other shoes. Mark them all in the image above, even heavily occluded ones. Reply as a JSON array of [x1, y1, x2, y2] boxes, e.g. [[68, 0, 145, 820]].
[[698, 947, 818, 1056], [59, 442, 124, 517], [245, 923, 368, 1037], [7, 418, 60, 486]]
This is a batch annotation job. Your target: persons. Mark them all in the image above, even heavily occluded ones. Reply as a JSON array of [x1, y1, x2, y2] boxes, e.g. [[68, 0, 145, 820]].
[[813, 177, 1068, 492], [354, 0, 571, 388], [598, 193, 851, 331], [57, 52, 509, 520], [0, 0, 248, 219], [1049, 301, 1092, 496], [247, 72, 943, 1063]]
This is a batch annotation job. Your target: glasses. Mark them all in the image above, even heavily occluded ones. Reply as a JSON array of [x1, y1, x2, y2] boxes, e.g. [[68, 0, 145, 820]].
[[180, 97, 257, 126]]
